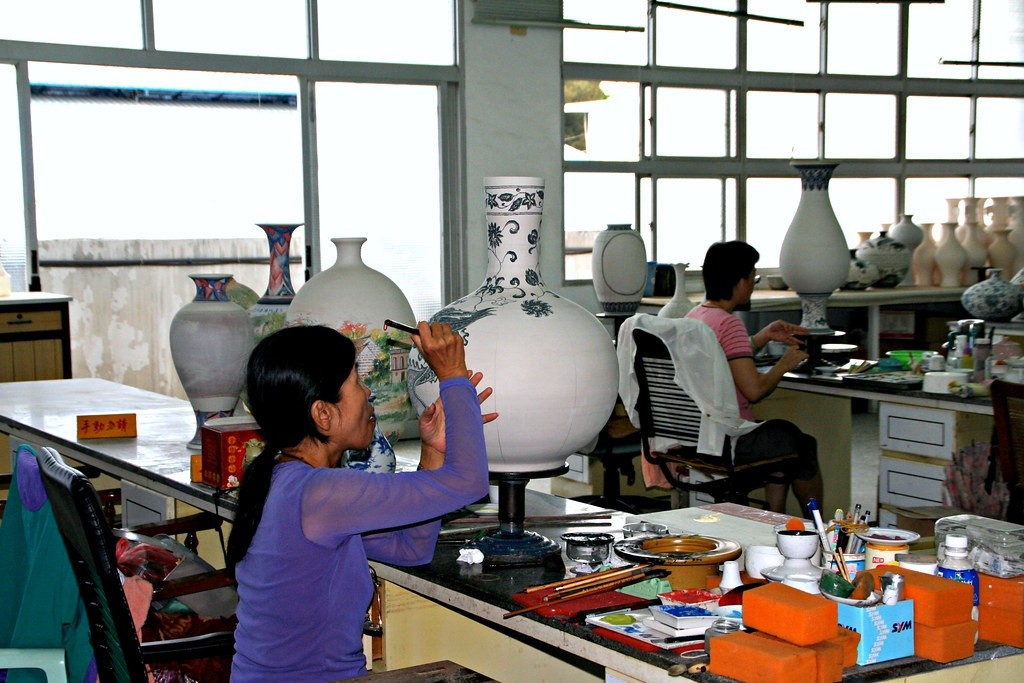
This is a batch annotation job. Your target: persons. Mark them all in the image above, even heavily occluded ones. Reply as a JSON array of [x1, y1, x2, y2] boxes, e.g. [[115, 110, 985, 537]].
[[684, 240, 824, 522], [227, 319, 499, 683]]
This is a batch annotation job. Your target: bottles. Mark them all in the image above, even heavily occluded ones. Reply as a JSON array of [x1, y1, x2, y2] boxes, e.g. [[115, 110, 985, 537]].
[[644, 262, 697, 317], [839, 195, 1024, 384], [236, 225, 302, 416], [934, 535, 980, 646], [287, 237, 417, 447], [704, 618, 743, 654], [778, 161, 850, 335], [338, 397, 396, 475], [170, 273, 253, 449], [592, 224, 646, 316], [408, 177, 618, 473]]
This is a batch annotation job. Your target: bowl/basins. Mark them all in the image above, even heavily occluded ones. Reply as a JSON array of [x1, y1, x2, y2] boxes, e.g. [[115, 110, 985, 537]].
[[744, 543, 786, 578], [922, 372, 969, 395], [559, 532, 616, 564], [820, 570, 855, 597], [776, 531, 819, 559], [765, 274, 787, 289], [885, 350, 927, 370]]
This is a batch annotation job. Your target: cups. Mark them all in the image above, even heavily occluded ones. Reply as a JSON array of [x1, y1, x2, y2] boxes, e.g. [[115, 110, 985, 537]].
[[821, 551, 865, 582], [622, 523, 667, 538], [864, 543, 910, 571]]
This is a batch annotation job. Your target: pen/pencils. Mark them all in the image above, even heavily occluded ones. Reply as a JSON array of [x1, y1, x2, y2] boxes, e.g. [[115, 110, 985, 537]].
[[807, 498, 871, 553]]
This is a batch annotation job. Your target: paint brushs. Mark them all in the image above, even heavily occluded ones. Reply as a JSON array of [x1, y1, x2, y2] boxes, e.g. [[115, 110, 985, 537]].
[[436, 514, 612, 545], [664, 634, 705, 643], [384, 319, 419, 335], [503, 562, 673, 619], [831, 547, 851, 582], [667, 659, 709, 677]]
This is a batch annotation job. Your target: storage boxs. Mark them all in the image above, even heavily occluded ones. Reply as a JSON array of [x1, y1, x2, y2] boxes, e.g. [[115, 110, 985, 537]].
[[200, 416, 266, 488], [840, 600, 915, 667]]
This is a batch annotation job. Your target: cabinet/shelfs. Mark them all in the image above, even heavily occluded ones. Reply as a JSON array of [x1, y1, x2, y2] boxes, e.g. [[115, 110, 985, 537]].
[[0, 299, 74, 474], [878, 401, 957, 539]]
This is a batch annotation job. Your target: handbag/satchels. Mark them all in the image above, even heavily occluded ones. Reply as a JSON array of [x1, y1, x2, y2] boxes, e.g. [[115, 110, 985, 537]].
[[112, 528, 239, 622]]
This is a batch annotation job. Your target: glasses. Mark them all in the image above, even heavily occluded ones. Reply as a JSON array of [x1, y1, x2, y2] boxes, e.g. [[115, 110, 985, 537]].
[[741, 274, 760, 285]]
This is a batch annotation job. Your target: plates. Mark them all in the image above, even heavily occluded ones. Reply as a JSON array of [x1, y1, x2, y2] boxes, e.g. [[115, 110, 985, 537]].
[[649, 604, 720, 628], [855, 529, 921, 546], [466, 503, 498, 515], [656, 592, 722, 607], [711, 605, 742, 623]]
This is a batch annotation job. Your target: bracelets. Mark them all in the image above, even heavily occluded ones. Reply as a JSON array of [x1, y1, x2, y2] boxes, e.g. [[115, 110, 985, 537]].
[[749, 336, 760, 351]]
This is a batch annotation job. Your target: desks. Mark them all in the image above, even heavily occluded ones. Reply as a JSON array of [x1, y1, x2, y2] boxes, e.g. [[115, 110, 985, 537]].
[[639, 287, 968, 360], [0, 377, 1023, 682]]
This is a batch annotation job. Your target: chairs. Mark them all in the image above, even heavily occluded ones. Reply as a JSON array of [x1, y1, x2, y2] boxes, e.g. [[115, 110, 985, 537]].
[[617, 315, 789, 507], [14, 445, 225, 626], [39, 445, 388, 683]]
